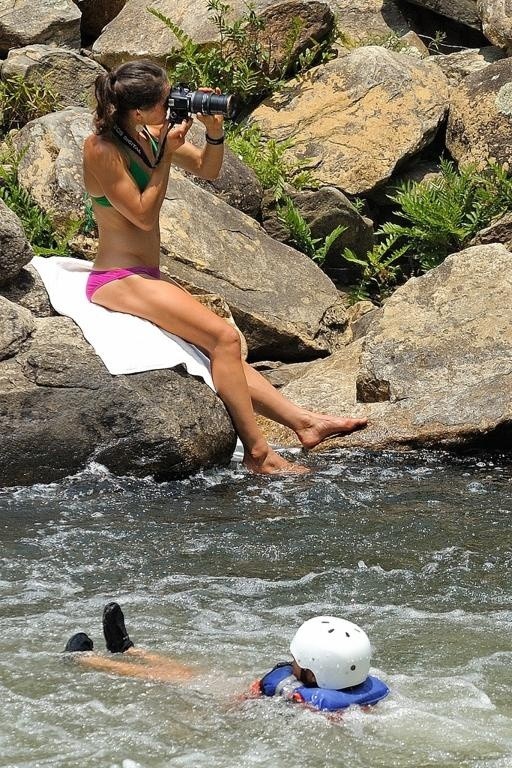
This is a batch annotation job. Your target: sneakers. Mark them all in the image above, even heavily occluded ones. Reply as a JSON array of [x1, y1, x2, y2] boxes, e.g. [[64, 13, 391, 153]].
[[66, 633, 93, 651], [103, 602, 133, 653]]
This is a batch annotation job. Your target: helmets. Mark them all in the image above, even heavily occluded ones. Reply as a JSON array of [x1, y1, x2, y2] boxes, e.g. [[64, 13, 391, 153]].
[[289, 616, 370, 690]]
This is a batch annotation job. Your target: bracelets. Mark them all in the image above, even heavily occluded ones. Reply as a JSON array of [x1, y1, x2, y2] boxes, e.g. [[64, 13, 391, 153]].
[[205, 133, 223, 145]]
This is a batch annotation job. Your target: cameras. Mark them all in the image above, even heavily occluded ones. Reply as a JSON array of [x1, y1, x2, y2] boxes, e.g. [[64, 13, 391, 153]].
[[168, 82, 235, 123]]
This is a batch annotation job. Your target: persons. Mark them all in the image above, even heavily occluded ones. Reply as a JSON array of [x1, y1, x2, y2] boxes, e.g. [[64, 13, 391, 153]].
[[64, 602, 390, 712], [85, 59, 367, 472]]
